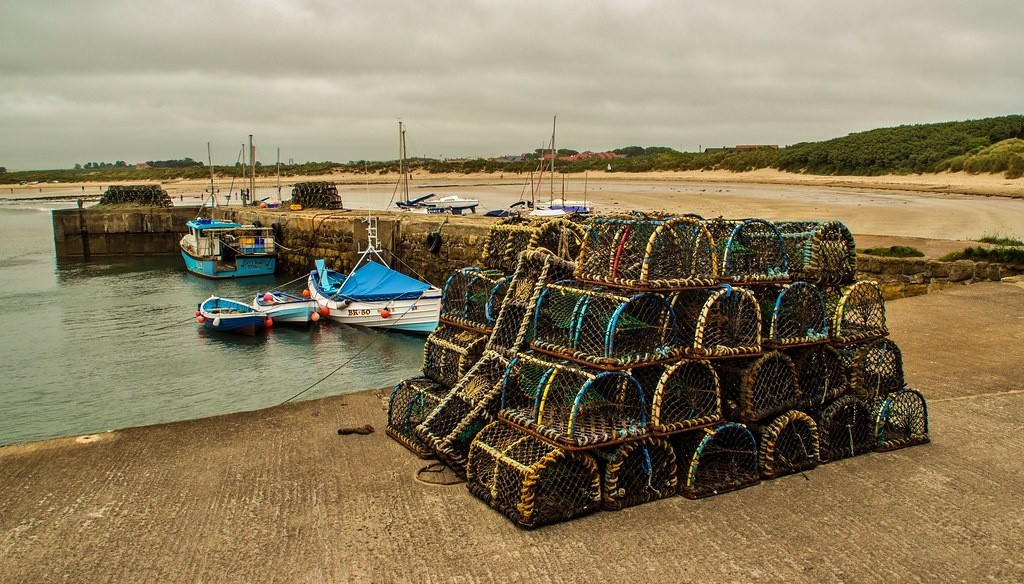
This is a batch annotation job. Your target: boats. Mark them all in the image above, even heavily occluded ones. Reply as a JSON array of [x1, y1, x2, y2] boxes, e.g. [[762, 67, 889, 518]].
[[395, 116, 593, 217], [196, 295, 272, 335], [303, 209, 444, 334], [253, 290, 322, 326], [177, 142, 277, 280]]
